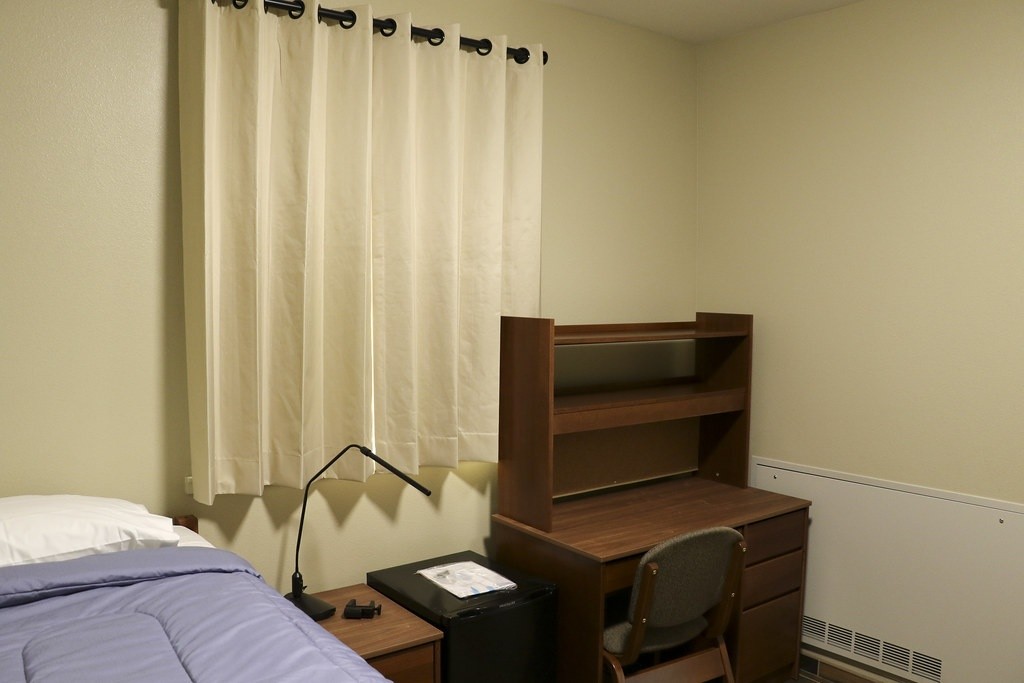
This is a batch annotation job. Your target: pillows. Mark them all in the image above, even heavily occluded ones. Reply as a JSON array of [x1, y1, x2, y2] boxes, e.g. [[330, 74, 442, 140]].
[[0, 494, 180, 568]]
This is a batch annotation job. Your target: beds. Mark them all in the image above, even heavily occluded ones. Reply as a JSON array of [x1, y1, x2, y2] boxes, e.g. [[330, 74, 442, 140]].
[[0, 494, 396, 683]]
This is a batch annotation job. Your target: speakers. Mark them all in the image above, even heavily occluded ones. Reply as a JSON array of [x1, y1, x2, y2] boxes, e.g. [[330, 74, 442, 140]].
[[363, 549, 559, 683]]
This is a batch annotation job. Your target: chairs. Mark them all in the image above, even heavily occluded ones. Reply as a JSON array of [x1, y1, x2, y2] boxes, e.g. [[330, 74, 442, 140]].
[[601, 526, 748, 683]]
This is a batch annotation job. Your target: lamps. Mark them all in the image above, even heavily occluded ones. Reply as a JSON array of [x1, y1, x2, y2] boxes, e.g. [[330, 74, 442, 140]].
[[284, 445, 431, 621]]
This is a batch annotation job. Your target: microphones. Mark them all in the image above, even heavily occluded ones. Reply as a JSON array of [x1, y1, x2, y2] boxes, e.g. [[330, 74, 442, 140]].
[[282, 445, 433, 621]]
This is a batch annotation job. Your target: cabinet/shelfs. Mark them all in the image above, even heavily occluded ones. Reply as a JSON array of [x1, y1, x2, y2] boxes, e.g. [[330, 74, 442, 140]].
[[490, 311, 811, 683], [367, 551, 559, 683]]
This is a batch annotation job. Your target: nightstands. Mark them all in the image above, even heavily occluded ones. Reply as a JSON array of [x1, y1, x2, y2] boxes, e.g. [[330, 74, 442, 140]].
[[308, 583, 444, 683]]
